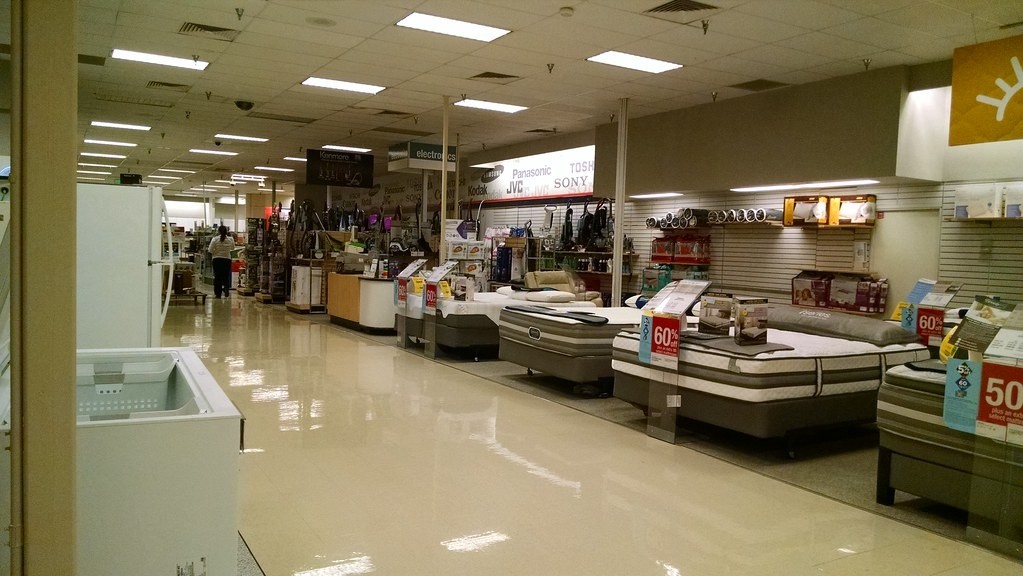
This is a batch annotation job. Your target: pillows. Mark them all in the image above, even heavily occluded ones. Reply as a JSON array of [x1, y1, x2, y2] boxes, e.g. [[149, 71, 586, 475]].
[[525, 288, 577, 302], [495, 284, 527, 302], [622, 293, 702, 317], [768, 306, 921, 349]]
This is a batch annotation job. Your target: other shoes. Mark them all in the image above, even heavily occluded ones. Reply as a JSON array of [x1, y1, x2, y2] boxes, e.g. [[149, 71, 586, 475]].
[[215, 295, 220, 298], [222, 287, 229, 297]]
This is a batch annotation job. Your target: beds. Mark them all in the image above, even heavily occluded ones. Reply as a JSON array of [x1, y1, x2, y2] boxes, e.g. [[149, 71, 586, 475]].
[[609, 303, 931, 458], [871, 358, 1023, 527], [394, 269, 605, 361], [498, 290, 701, 397]]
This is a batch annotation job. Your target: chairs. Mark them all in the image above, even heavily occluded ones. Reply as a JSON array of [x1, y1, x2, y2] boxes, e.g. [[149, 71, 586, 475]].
[[524, 268, 604, 308]]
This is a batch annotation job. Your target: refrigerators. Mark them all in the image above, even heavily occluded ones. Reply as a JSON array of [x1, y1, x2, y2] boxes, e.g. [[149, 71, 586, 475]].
[[76, 183, 174, 350], [0, 346, 245, 576]]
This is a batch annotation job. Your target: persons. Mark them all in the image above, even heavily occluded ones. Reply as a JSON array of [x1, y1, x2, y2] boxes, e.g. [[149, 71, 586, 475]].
[[796, 288, 815, 307], [976, 306, 995, 319], [208, 226, 236, 299]]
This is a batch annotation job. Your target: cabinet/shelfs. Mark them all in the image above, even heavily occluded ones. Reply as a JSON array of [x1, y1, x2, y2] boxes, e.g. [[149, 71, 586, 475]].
[[524, 234, 641, 280]]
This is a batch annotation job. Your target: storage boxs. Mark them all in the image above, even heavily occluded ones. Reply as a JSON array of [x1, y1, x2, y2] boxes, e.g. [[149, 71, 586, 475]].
[[651, 239, 674, 263], [697, 291, 733, 337], [733, 293, 770, 345], [674, 241, 710, 267], [786, 265, 889, 317], [407, 212, 494, 300]]
